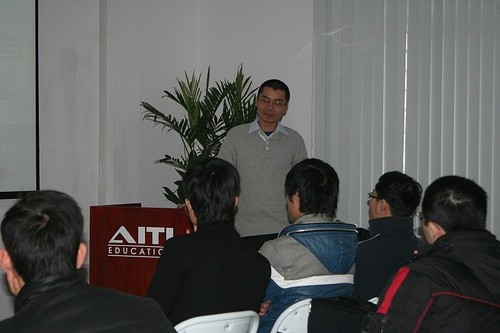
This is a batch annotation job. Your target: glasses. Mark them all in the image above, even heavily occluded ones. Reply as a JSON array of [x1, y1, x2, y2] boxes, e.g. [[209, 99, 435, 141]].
[[368, 192, 380, 200], [419, 212, 426, 221], [258, 97, 286, 107]]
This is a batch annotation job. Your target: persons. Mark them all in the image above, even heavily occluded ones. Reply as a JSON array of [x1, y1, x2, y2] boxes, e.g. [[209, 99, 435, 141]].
[[215, 77, 309, 250], [354, 170, 423, 309], [145, 157, 272, 328], [258, 158, 359, 332], [0, 189, 177, 333], [362, 175, 500, 333]]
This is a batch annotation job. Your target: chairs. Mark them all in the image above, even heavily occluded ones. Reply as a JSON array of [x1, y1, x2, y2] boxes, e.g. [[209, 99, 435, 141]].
[[271, 298, 311, 332], [174, 310, 260, 332]]
